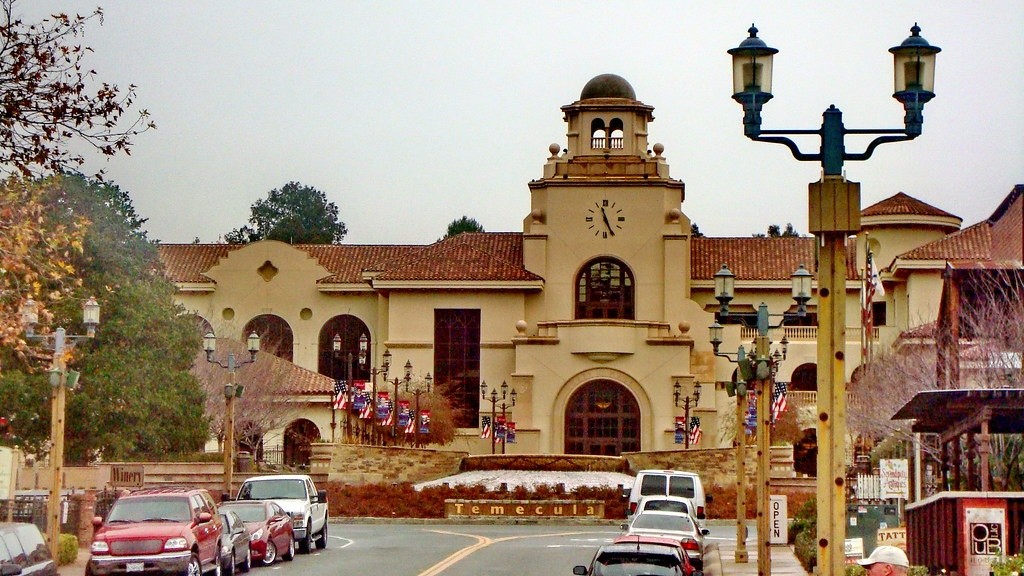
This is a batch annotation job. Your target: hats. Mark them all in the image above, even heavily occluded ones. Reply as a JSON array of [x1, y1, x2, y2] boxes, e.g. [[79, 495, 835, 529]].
[[857, 546, 909, 569]]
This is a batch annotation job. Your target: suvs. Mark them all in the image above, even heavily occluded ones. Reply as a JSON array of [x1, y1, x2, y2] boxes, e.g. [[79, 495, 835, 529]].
[[83, 486, 223, 576], [235, 474, 329, 554]]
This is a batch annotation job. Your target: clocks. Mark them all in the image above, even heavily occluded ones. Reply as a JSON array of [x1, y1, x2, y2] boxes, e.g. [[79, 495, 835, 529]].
[[585, 200, 625, 238]]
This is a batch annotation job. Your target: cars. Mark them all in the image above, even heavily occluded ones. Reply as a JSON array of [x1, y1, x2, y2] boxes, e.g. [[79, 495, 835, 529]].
[[624, 496, 705, 524], [620, 510, 710, 570], [611, 535, 698, 576], [571, 542, 705, 576], [0, 521, 63, 576], [216, 509, 252, 576], [216, 501, 296, 566]]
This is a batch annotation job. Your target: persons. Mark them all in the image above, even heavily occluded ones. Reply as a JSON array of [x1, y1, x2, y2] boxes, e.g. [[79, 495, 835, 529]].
[[846, 486, 859, 504], [856, 546, 909, 576], [882, 498, 897, 515]]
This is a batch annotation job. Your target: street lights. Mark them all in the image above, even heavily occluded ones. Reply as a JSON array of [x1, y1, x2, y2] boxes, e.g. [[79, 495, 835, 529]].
[[17, 290, 102, 569], [708, 318, 784, 564], [489, 387, 517, 454], [479, 378, 508, 454], [359, 349, 392, 443], [333, 330, 369, 441], [713, 261, 815, 576], [727, 20, 943, 576], [673, 380, 704, 453], [404, 371, 433, 448], [380, 360, 413, 446], [202, 329, 261, 501]]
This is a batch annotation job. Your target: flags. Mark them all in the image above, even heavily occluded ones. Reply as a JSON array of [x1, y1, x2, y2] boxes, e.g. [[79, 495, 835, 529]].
[[744, 390, 757, 435], [398, 407, 416, 433], [494, 416, 516, 444], [419, 413, 430, 433], [858, 243, 886, 355], [771, 382, 788, 428], [480, 416, 491, 439], [352, 380, 371, 419], [689, 416, 700, 445], [332, 380, 346, 410], [674, 417, 685, 444], [378, 392, 394, 425]]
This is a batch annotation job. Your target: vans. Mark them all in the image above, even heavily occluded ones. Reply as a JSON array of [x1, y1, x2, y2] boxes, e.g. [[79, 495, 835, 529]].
[[624, 468, 714, 535]]
